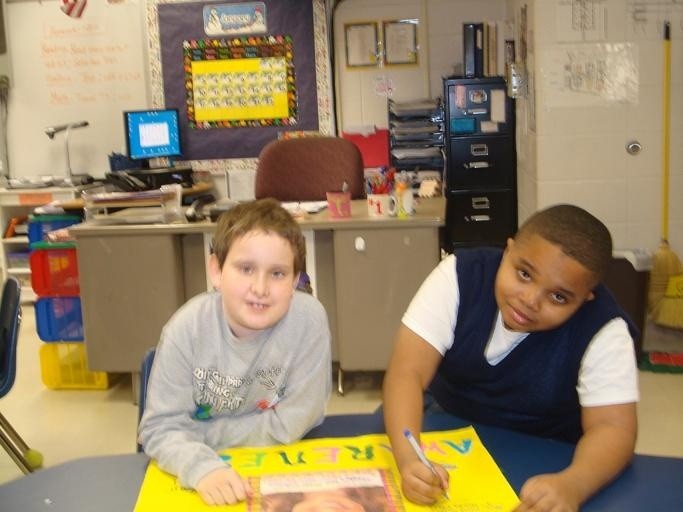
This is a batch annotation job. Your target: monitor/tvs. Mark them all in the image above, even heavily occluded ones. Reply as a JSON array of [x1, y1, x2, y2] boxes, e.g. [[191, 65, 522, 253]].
[[122, 107, 183, 169]]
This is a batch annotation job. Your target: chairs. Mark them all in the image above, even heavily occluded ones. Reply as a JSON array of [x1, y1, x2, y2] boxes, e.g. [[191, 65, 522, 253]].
[[254, 138, 366, 200], [0, 277, 45, 476]]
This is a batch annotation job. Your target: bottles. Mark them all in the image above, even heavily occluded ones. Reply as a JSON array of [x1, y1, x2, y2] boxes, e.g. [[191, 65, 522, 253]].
[[395, 169, 411, 221]]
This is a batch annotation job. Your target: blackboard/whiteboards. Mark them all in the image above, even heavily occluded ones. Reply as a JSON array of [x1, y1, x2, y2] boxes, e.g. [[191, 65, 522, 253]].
[[1, 0, 156, 189]]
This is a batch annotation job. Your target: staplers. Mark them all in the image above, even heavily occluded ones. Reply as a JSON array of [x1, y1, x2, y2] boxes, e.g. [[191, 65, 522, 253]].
[[186, 195, 215, 221]]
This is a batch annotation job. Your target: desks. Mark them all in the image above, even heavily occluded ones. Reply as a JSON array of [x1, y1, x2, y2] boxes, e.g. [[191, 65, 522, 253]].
[[0, 411, 683, 512], [70, 198, 447, 405]]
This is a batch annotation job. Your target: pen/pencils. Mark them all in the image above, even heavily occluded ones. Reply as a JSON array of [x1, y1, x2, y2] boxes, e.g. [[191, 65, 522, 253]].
[[365, 165, 417, 194], [343, 177, 348, 192], [404, 431, 449, 500]]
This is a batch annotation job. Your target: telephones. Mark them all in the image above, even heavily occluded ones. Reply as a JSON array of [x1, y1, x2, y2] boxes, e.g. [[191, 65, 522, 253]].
[[105, 172, 149, 192]]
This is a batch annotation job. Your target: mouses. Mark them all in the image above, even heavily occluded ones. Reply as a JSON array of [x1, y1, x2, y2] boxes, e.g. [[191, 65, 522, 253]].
[[181, 182, 192, 188]]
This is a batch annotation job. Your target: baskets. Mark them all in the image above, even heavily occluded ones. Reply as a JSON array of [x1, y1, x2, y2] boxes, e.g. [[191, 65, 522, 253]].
[[27, 215, 123, 389]]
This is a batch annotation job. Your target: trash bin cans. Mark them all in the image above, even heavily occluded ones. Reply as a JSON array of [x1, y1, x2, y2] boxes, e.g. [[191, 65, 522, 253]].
[[599, 248, 652, 365]]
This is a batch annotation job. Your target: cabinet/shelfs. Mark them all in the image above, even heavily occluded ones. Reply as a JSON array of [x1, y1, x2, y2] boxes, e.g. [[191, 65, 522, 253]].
[[391, 76, 518, 253], [0, 172, 214, 303]]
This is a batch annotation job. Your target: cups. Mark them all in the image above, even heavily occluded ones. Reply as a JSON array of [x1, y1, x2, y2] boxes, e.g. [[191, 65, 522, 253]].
[[159, 184, 183, 224], [326, 190, 351, 221], [366, 192, 397, 220]]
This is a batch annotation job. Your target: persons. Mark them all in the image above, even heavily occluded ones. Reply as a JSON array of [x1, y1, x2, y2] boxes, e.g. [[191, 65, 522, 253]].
[[381, 204, 639, 512], [136, 197, 333, 507]]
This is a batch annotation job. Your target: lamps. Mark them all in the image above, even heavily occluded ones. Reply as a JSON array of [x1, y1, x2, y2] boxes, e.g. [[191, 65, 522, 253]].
[[45, 119, 94, 185]]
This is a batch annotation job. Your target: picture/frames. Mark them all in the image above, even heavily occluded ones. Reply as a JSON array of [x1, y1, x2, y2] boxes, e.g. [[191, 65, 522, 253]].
[[145, 2, 337, 174]]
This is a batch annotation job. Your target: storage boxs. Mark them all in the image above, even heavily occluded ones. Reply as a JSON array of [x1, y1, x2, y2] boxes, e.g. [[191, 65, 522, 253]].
[[28, 214, 121, 390]]
